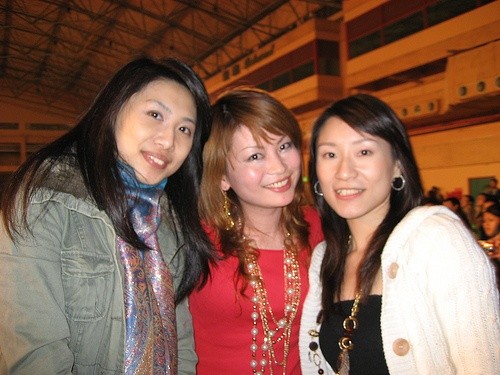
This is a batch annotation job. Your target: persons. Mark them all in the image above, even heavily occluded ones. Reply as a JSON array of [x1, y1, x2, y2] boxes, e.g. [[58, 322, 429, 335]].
[[442, 197, 470, 228], [484, 184, 498, 201], [186, 85, 325, 375], [1, 55, 216, 374], [482, 203, 500, 264], [475, 193, 492, 218], [460, 195, 475, 225], [487, 177, 500, 198], [481, 198, 499, 215], [299, 93, 500, 375]]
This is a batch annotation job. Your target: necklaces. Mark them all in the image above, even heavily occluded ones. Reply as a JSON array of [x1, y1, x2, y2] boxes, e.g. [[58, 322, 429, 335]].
[[240, 232, 301, 375], [309, 236, 363, 375]]
